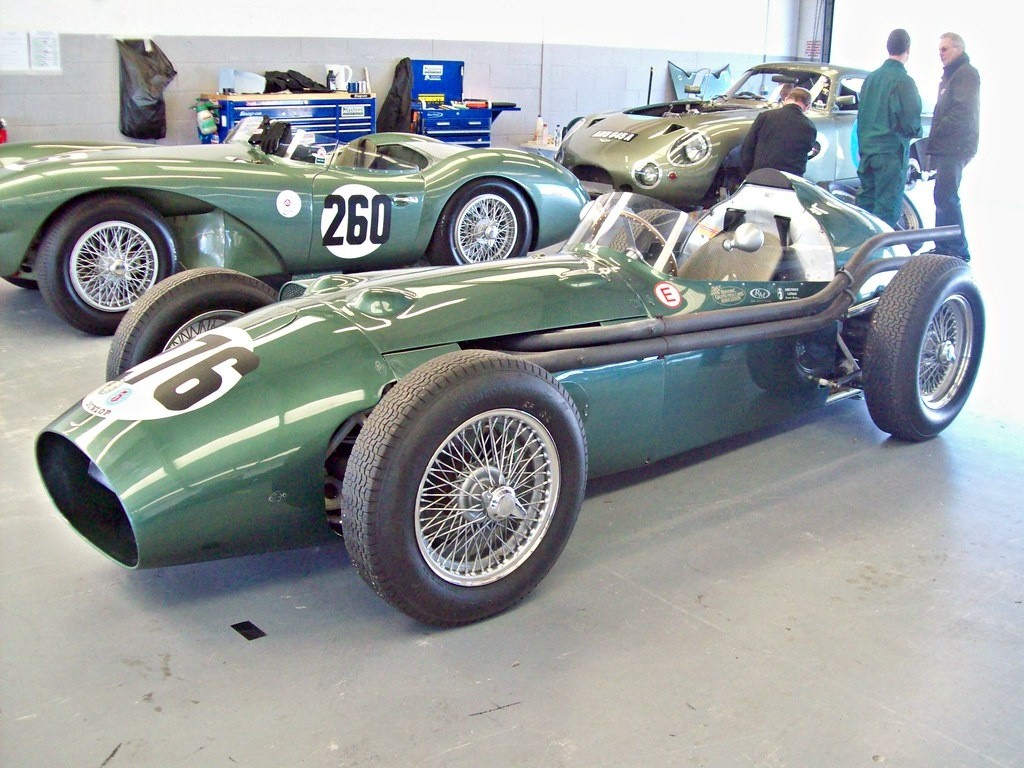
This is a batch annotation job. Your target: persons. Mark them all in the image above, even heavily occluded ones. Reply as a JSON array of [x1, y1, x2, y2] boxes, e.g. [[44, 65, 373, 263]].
[[740, 87, 818, 180], [852, 29, 925, 227], [924, 31, 981, 263]]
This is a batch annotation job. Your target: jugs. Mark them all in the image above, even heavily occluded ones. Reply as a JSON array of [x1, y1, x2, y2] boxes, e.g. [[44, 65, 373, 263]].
[[325, 65, 352, 91]]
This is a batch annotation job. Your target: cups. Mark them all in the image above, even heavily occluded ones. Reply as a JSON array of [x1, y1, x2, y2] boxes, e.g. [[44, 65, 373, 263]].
[[346, 83, 359, 92]]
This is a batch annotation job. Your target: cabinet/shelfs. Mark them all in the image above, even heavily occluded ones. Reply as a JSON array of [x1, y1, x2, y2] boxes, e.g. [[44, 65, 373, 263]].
[[400, 58, 521, 149]]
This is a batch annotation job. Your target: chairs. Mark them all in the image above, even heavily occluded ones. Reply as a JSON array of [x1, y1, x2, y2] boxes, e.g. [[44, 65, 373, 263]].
[[333, 138, 377, 169], [671, 167, 835, 282]]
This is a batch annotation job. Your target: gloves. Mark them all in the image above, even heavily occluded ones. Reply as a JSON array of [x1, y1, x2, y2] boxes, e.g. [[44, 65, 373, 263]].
[[261, 122, 292, 153]]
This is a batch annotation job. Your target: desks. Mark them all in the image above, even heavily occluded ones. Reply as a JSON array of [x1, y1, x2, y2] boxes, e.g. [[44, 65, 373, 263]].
[[199, 91, 376, 153]]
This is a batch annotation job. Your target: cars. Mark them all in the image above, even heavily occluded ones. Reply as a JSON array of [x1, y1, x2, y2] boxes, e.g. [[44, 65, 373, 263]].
[[1, 117, 591, 335], [34, 167, 987, 630], [555, 61, 936, 213]]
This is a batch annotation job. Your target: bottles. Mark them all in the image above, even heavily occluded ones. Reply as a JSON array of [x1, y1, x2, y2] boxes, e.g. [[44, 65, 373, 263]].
[[197, 105, 217, 135], [554, 125, 561, 148], [327, 70, 335, 90], [543, 124, 547, 144], [535, 115, 543, 144]]
